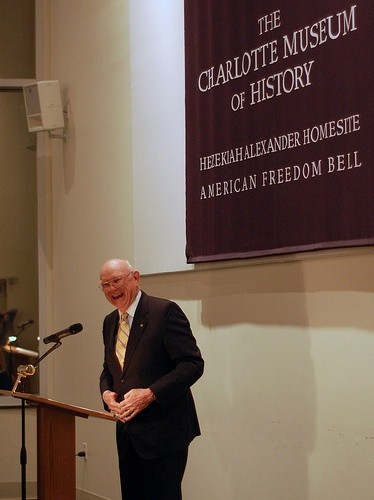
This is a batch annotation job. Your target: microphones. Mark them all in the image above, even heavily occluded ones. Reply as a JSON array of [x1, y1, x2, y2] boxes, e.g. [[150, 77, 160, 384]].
[[44, 323, 84, 344], [2, 346, 40, 358]]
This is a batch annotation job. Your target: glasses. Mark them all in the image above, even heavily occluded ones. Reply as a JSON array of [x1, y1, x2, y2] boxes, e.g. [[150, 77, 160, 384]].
[[98, 269, 134, 293]]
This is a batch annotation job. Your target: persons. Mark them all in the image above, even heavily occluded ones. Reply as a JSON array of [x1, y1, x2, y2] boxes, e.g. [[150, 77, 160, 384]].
[[98, 258, 205, 500], [0, 307, 18, 390]]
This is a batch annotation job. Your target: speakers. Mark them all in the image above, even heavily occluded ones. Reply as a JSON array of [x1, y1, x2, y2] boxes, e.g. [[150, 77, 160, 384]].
[[23, 79, 65, 132]]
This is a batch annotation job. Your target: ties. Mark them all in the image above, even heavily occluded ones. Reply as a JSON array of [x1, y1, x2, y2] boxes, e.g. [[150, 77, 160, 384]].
[[114, 312, 131, 372]]
